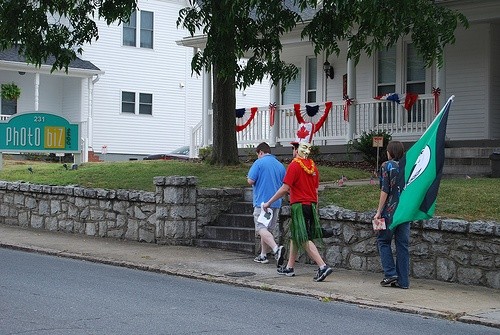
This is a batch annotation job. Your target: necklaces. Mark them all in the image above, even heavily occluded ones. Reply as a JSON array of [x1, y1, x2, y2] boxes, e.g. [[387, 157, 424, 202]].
[[294, 157, 314, 174]]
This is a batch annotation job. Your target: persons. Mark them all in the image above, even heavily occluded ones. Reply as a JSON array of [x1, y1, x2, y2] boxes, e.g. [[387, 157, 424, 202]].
[[263, 122, 333, 282], [373, 141, 410, 289], [246, 142, 286, 268]]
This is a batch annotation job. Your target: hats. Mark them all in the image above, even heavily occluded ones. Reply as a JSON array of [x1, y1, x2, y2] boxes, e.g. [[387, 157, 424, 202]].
[[291, 122, 316, 145]]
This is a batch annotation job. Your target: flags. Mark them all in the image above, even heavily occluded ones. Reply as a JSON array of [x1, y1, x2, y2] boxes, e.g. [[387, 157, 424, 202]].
[[387, 100, 451, 230]]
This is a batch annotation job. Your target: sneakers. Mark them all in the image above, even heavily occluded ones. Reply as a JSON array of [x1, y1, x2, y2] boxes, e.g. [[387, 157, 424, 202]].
[[395, 282, 410, 288], [254, 254, 269, 263], [277, 265, 296, 276], [381, 275, 398, 285], [312, 263, 332, 282], [273, 245, 285, 269]]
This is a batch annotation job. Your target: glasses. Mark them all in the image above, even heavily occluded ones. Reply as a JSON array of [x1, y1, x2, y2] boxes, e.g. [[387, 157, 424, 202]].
[[292, 147, 297, 151]]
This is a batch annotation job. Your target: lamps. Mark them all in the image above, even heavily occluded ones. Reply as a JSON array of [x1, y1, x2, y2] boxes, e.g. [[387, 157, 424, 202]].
[[323, 58, 334, 79]]
